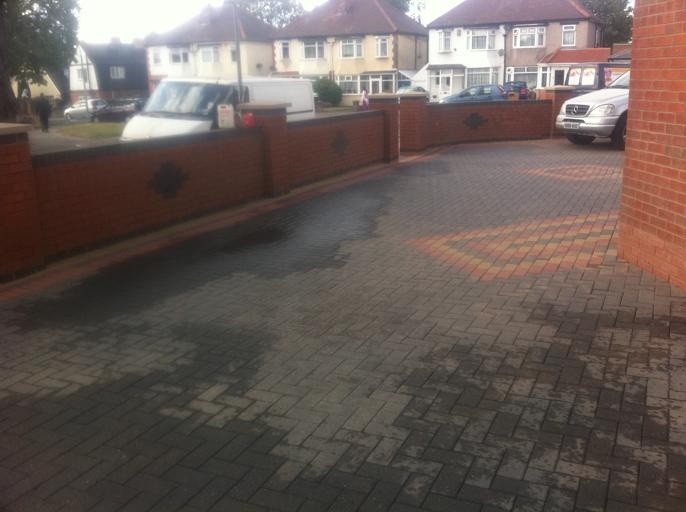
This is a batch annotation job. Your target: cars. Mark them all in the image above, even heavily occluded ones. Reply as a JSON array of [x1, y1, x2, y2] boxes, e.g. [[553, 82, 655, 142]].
[[395, 85, 431, 103], [439, 83, 506, 103], [555, 68, 630, 150]]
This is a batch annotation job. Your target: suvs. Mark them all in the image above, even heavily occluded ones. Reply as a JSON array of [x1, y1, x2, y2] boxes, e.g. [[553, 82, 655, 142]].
[[90, 97, 143, 124], [505, 80, 529, 100]]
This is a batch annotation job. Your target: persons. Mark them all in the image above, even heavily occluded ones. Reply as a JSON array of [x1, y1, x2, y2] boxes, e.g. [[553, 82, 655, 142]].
[[33, 92, 52, 134], [527, 88, 537, 100], [358, 88, 370, 112]]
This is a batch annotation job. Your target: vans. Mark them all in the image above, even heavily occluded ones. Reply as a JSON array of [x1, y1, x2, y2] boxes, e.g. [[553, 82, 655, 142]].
[[121, 73, 318, 144], [63, 97, 109, 121]]
